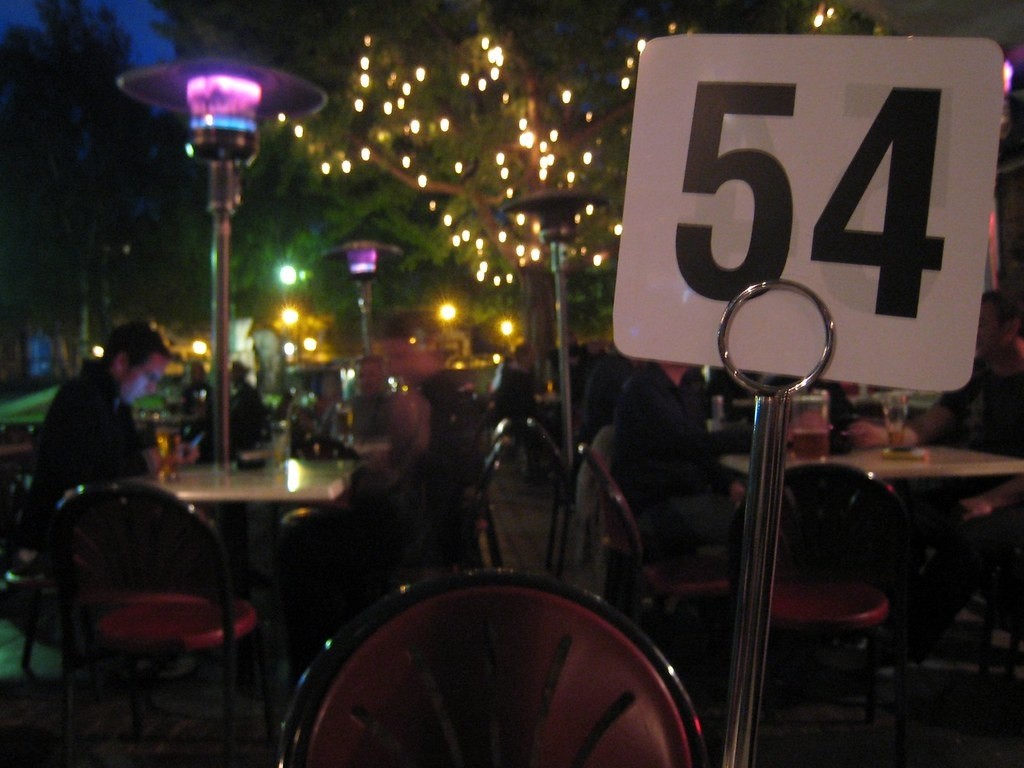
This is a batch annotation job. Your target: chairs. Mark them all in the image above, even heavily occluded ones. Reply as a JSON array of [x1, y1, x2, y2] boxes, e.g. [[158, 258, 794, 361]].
[[0, 367, 1024, 768]]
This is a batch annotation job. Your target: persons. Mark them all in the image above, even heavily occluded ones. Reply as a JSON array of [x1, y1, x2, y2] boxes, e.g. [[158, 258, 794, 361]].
[[847, 289, 1024, 665], [494, 338, 621, 479], [17, 322, 202, 681], [613, 362, 796, 514], [746, 370, 857, 456], [229, 362, 253, 461], [352, 355, 393, 445], [278, 310, 490, 665], [180, 362, 212, 463]]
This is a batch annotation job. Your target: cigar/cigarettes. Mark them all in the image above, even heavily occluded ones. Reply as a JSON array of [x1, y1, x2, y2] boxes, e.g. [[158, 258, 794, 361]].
[[841, 431, 854, 436]]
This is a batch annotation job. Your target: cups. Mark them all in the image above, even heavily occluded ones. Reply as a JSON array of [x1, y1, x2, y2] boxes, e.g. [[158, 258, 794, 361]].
[[156, 426, 179, 480], [881, 398, 907, 447], [270, 423, 289, 470], [791, 394, 829, 461]]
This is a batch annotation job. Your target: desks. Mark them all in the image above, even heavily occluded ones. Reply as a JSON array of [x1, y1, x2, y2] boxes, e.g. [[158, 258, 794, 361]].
[[729, 392, 932, 419], [63, 451, 358, 697], [132, 411, 208, 446], [711, 434, 1024, 690]]
